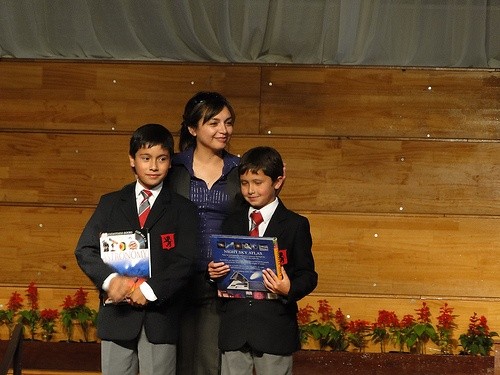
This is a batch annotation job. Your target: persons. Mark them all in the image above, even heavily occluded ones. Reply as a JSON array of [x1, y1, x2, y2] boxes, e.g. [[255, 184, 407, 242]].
[[209, 146, 318, 375], [163, 91, 287, 375], [74, 123, 200, 375]]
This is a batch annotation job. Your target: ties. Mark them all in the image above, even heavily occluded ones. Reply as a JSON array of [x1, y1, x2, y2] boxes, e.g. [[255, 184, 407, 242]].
[[249, 213, 264, 237], [138, 190, 152, 230]]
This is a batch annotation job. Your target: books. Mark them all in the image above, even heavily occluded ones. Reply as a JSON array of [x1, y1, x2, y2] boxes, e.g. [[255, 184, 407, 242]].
[[211, 235, 283, 300], [100, 228, 152, 303]]
